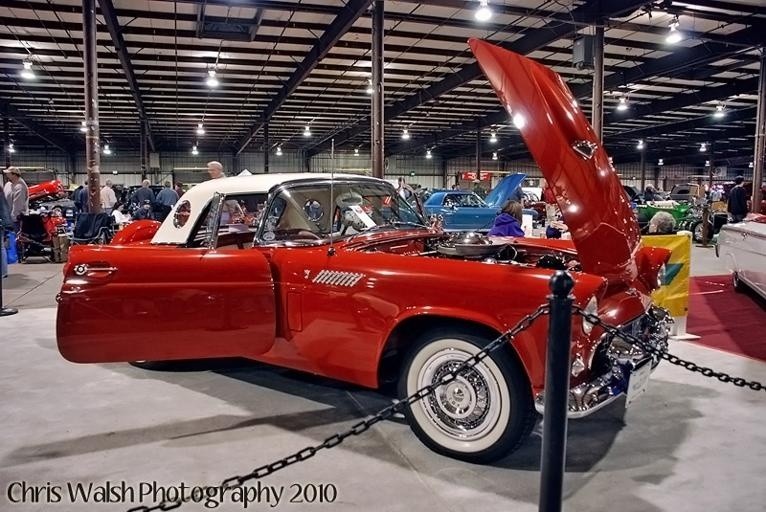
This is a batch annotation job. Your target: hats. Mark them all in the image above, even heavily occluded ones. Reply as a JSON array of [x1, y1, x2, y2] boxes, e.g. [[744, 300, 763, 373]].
[[2, 166, 20, 176]]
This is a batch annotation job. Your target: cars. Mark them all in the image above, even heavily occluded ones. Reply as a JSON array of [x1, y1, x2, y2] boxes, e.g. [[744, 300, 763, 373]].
[[55, 36, 674, 464], [632, 196, 727, 244], [714, 216, 766, 300], [380, 172, 538, 230], [523, 191, 546, 213]]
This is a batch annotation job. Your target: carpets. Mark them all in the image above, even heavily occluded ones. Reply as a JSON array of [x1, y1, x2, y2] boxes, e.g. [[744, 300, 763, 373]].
[[680, 274, 766, 361]]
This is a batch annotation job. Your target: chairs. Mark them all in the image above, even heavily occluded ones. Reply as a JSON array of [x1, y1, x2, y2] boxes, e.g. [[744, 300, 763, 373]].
[[641, 230, 703, 342]]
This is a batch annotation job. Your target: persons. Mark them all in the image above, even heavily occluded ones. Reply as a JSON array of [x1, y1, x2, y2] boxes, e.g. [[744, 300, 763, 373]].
[[643, 175, 766, 235], [396, 175, 570, 239], [0, 160, 242, 277]]
